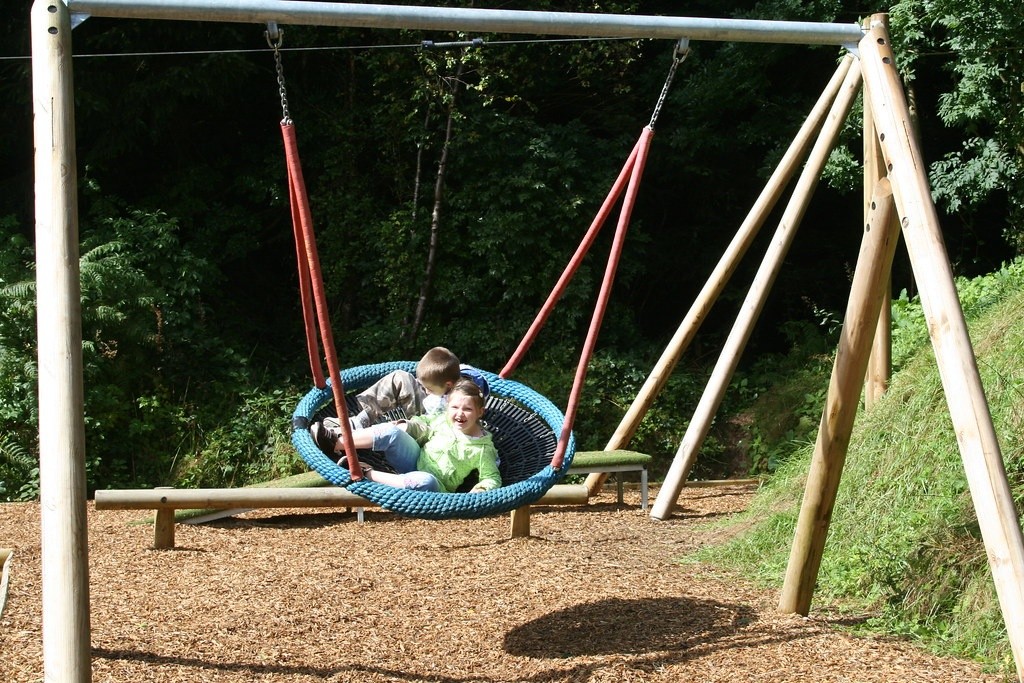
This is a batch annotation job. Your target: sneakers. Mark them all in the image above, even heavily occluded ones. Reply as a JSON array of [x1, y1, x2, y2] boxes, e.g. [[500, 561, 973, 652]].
[[337, 456, 372, 477], [310, 421, 342, 460]]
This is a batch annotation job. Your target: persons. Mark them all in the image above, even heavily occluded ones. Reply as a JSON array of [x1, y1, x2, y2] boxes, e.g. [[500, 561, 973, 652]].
[[311, 379, 502, 495], [322, 347, 491, 444]]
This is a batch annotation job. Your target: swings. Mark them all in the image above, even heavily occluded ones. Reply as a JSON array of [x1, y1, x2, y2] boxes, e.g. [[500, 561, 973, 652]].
[[262, 22, 691, 522]]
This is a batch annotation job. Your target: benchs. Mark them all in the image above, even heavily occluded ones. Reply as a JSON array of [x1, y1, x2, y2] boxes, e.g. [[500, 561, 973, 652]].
[[151, 450, 650, 526]]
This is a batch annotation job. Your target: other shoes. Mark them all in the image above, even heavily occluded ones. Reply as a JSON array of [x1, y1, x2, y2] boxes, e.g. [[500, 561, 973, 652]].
[[322, 416, 354, 438], [357, 450, 382, 465]]
